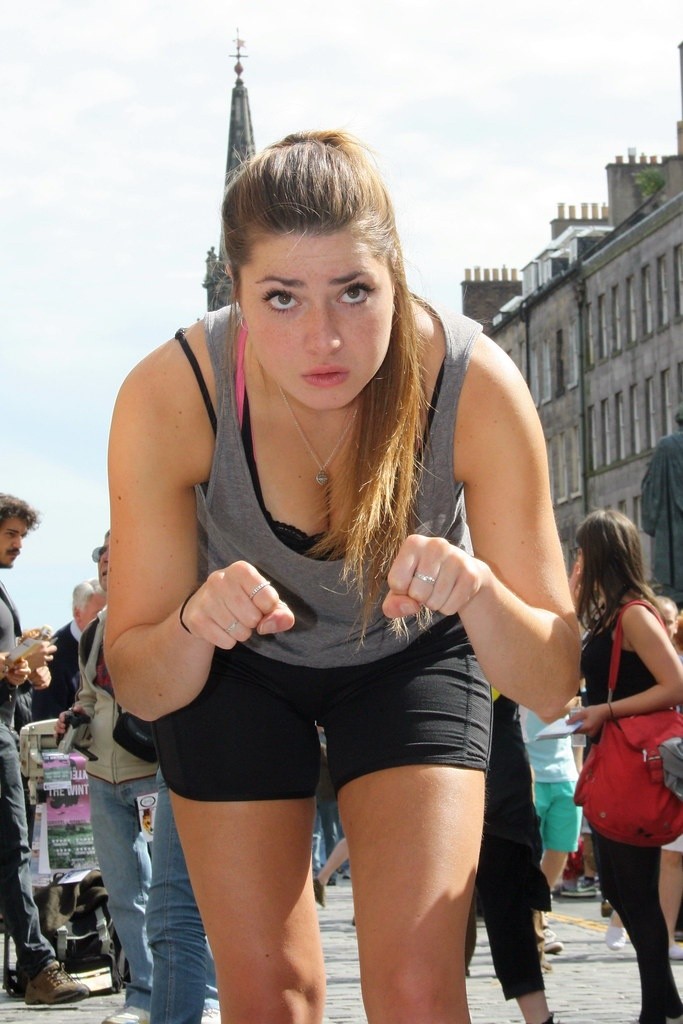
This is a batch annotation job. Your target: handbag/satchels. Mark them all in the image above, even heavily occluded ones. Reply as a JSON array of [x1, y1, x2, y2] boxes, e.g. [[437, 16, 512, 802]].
[[113, 703, 158, 763], [573, 599, 683, 848]]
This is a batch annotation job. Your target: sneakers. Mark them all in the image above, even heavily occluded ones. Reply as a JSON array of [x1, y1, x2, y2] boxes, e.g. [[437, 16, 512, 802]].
[[101, 1006, 151, 1024], [201, 1009, 221, 1024], [24, 961, 90, 1005]]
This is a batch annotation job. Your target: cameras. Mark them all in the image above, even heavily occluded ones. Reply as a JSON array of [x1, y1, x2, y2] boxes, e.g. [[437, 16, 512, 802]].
[[55, 711, 98, 762]]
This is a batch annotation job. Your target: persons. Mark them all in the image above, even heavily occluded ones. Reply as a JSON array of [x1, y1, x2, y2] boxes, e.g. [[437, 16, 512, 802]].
[[0, 495, 357, 1024], [638, 409, 682, 608], [104, 130, 583, 1024], [464, 509, 683, 1024]]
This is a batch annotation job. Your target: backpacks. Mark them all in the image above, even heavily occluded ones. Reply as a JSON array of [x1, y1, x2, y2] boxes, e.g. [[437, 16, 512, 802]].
[[34, 868, 131, 997]]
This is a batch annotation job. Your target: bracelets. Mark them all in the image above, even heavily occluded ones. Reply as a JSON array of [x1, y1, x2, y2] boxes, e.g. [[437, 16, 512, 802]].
[[180, 586, 202, 635], [607, 702, 615, 720]]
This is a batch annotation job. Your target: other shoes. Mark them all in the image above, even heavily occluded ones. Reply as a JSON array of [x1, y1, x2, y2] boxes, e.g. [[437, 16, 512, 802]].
[[560, 881, 601, 898], [606, 910, 627, 951], [601, 899, 614, 917], [313, 879, 326, 908], [542, 928, 564, 954], [343, 876, 349, 879], [668, 947, 683, 960], [327, 878, 336, 885]]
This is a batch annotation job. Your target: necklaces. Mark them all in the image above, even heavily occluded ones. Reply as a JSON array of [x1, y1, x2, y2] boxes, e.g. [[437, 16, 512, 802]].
[[278, 385, 361, 487]]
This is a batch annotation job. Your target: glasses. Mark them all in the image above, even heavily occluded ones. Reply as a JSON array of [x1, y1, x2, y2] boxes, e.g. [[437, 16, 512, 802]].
[[92, 546, 107, 562]]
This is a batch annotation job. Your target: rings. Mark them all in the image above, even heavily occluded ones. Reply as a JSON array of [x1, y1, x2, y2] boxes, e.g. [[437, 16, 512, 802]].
[[4, 664, 10, 673], [224, 619, 240, 635], [249, 581, 270, 599], [415, 573, 437, 585]]
